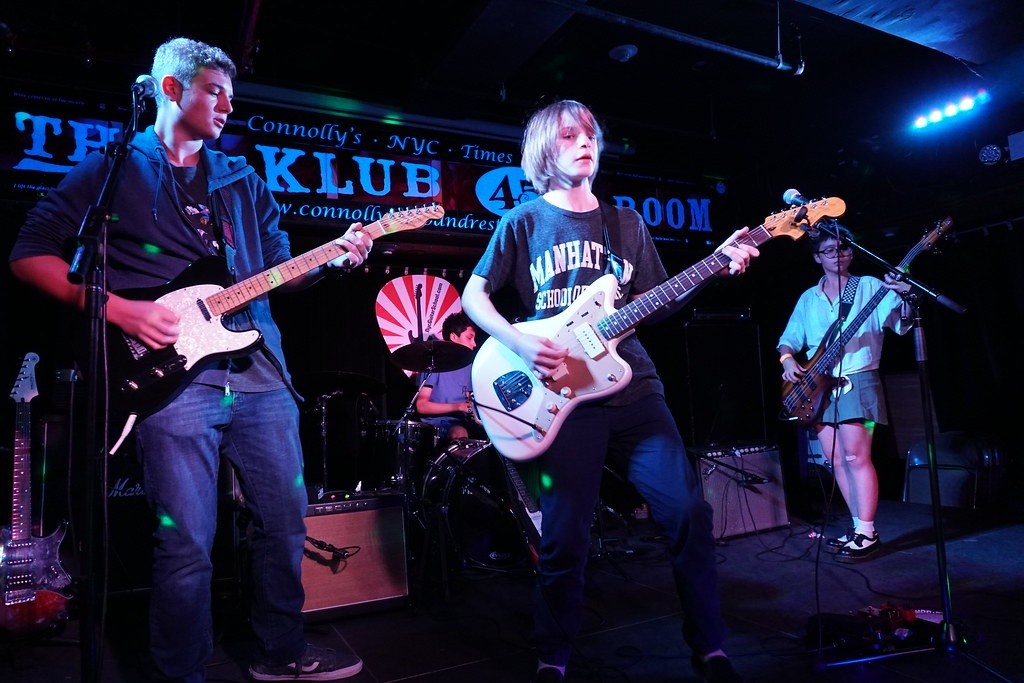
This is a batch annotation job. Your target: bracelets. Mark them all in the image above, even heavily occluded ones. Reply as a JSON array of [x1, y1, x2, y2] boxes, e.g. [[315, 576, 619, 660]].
[[779, 352, 792, 364]]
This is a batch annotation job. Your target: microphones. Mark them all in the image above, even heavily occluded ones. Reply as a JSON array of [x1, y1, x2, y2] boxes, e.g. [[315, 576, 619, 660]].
[[743, 476, 770, 484], [783, 190, 838, 223], [369, 401, 382, 419], [132, 74, 161, 97], [332, 549, 350, 561]]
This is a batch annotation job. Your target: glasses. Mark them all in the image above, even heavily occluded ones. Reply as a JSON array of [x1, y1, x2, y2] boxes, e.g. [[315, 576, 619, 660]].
[[818, 244, 852, 258]]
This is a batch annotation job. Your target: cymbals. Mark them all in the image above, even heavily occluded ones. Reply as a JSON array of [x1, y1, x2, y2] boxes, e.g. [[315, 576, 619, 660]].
[[387, 340, 474, 373], [301, 370, 380, 398]]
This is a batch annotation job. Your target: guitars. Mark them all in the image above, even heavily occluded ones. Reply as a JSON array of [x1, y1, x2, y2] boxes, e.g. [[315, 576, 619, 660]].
[[470, 194, 848, 465], [93, 201, 446, 421], [1, 350, 75, 640], [780, 214, 955, 427]]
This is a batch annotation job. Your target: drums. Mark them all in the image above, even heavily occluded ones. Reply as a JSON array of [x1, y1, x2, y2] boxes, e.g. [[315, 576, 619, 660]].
[[355, 420, 438, 493], [418, 438, 518, 546]]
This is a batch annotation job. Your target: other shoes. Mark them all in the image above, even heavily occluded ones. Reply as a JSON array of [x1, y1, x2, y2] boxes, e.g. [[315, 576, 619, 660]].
[[529, 657, 568, 683], [691, 651, 743, 683]]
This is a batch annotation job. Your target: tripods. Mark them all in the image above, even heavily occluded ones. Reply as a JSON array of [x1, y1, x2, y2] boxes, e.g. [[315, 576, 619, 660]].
[[812, 219, 1014, 683]]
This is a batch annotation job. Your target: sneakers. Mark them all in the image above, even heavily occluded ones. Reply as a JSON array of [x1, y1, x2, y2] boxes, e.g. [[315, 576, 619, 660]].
[[248, 640, 363, 681], [826, 526, 856, 548], [840, 529, 881, 558]]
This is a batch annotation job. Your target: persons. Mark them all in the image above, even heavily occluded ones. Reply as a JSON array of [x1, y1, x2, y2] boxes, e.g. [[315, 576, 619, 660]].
[[774, 218, 918, 565], [413, 312, 489, 450], [461, 100, 761, 681], [8, 37, 375, 682]]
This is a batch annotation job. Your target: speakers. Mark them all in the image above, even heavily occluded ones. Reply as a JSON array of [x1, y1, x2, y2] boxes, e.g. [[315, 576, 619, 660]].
[[86, 322, 237, 597], [300, 488, 409, 616], [685, 445, 790, 541], [685, 320, 768, 447]]
